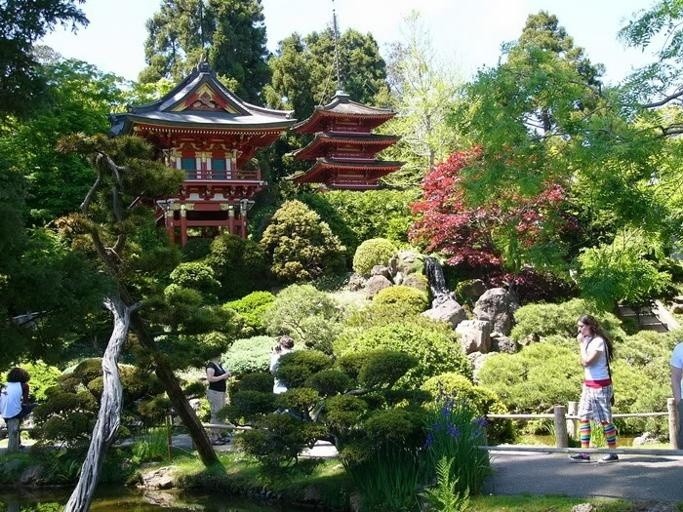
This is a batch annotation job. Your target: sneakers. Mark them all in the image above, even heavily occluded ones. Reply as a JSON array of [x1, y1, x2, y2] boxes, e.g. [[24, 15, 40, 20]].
[[598, 455, 619, 463], [214, 437, 230, 445], [569, 454, 591, 463]]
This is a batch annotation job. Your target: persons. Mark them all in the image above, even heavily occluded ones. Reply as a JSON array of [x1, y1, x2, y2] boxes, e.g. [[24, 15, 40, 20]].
[[570, 314, 618, 462], [271, 336, 295, 394], [1, 367, 35, 431], [672, 342, 683, 448], [207, 355, 230, 446]]
